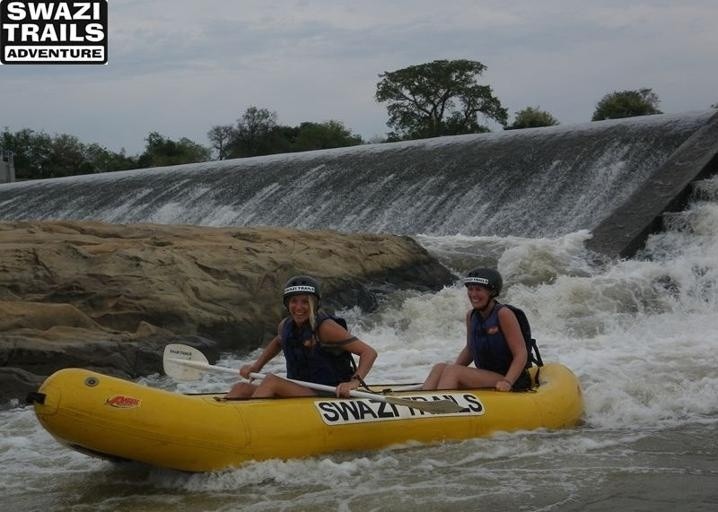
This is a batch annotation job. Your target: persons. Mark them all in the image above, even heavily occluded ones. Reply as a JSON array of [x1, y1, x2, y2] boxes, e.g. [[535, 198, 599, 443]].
[[225, 275, 377, 399], [422, 268, 532, 392]]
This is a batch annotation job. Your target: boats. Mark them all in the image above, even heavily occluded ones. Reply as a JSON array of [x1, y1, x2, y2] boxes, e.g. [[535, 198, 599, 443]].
[[25, 363, 586, 473]]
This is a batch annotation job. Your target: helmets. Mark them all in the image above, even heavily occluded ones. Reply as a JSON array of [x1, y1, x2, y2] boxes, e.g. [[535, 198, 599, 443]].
[[464, 267, 503, 296], [283, 275, 322, 309]]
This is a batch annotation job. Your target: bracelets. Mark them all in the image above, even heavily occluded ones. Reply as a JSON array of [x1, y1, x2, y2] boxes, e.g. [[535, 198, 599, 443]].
[[503, 378, 513, 388]]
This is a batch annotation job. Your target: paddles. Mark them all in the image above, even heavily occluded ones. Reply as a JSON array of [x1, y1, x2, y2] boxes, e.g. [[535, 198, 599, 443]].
[[163, 344, 464, 414]]
